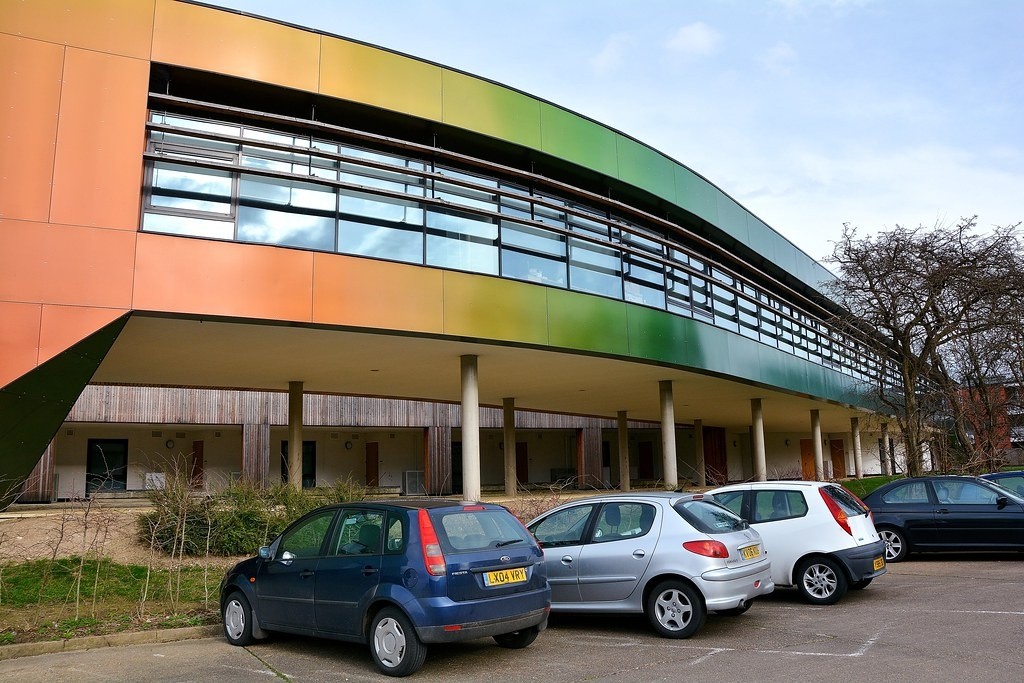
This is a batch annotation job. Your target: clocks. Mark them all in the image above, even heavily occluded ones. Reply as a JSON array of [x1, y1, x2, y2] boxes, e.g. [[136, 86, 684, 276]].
[[166, 440, 174, 448], [345, 441, 352, 449]]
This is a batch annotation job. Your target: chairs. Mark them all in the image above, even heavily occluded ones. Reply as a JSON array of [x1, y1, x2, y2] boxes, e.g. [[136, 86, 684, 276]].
[[769, 493, 787, 519], [938, 487, 952, 504], [604, 505, 622, 536], [360, 524, 380, 551], [636, 510, 654, 537]]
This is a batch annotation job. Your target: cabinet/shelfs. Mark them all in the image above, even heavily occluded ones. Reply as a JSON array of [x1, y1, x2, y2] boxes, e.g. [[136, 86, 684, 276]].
[[823, 460, 834, 479], [629, 465, 638, 480], [230, 471, 242, 497], [143, 472, 166, 490], [402, 469, 426, 496], [551, 468, 575, 490], [602, 466, 611, 489]]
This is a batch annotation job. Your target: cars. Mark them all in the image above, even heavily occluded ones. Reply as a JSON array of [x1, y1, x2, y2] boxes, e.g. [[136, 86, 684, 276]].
[[978, 471, 1024, 496], [860, 473, 1024, 561], [695, 481, 885, 603], [514, 459, 774, 636], [217, 496, 551, 676]]
